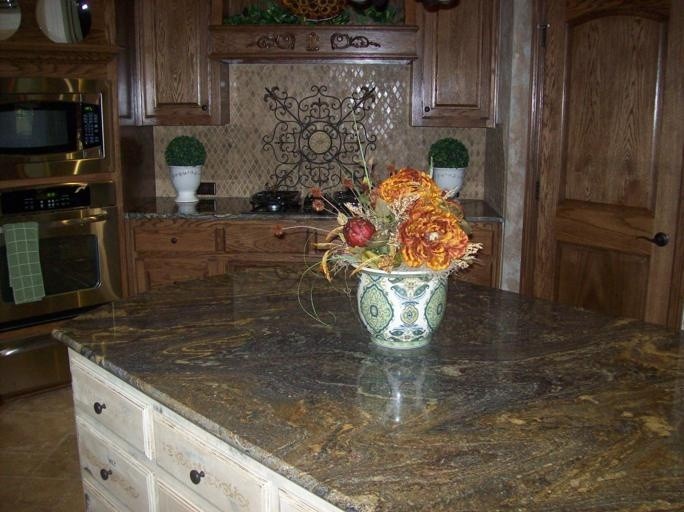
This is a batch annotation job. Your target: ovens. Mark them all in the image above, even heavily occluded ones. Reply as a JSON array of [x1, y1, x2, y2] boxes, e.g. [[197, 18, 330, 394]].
[[0, 182, 126, 325]]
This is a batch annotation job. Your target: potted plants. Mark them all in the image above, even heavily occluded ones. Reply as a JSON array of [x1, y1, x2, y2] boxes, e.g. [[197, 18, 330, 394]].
[[427, 137, 469, 199], [163, 135, 206, 203]]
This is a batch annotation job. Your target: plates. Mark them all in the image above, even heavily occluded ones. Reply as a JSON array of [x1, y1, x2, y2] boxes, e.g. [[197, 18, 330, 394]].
[[1, 1, 26, 43], [36, 0, 93, 47]]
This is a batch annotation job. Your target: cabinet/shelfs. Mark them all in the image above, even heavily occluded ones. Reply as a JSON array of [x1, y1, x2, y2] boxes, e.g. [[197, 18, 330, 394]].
[[404, 0, 499, 128], [133, 0, 231, 126], [450, 222, 500, 291], [220, 222, 355, 274], [125, 218, 220, 298], [65, 349, 154, 512], [154, 399, 277, 512]]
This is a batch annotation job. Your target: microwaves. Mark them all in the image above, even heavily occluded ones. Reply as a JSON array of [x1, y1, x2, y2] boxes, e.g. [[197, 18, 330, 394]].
[[1, 74, 116, 182]]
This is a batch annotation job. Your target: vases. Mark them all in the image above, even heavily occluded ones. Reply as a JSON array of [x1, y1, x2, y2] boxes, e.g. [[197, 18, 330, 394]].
[[346, 258, 447, 350]]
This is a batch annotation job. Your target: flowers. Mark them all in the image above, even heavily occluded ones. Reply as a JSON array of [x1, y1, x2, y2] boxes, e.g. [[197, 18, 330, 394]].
[[272, 102, 486, 338]]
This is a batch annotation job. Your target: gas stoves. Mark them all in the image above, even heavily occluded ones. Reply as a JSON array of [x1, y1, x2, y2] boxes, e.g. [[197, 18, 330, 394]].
[[243, 198, 366, 215]]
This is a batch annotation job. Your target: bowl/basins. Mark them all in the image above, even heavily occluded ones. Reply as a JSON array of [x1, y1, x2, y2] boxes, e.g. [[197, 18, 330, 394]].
[[252, 190, 301, 202]]
